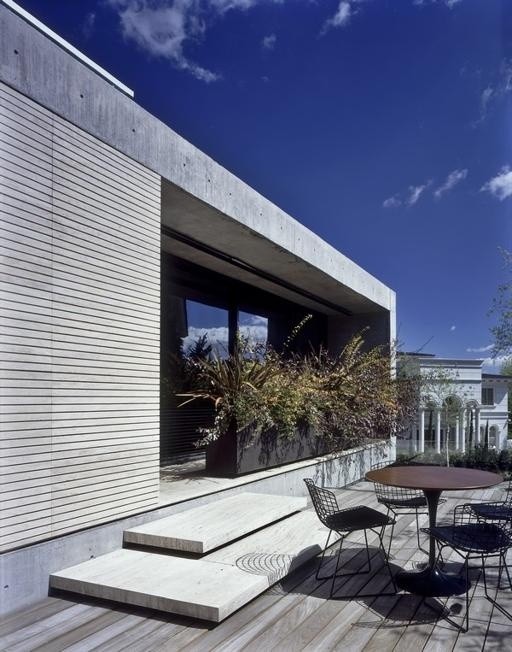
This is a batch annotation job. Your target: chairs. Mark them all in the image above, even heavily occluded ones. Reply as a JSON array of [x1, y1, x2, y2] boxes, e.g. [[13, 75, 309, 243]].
[[302, 457, 512, 634]]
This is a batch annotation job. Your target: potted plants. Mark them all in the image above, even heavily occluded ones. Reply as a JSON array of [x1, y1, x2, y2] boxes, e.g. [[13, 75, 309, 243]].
[[173, 313, 439, 478]]
